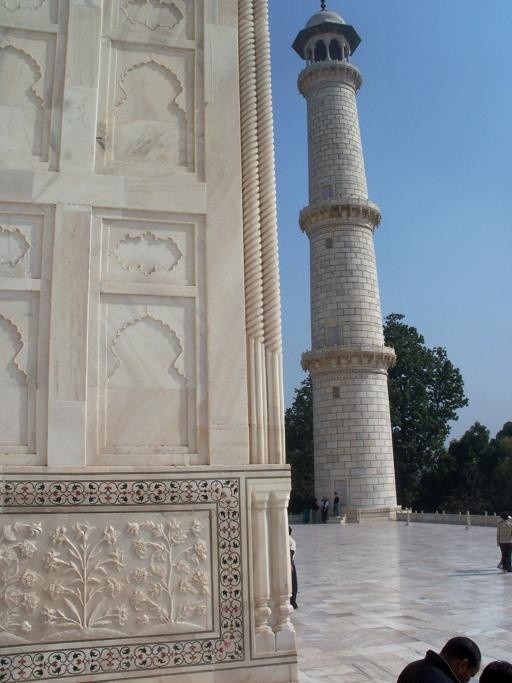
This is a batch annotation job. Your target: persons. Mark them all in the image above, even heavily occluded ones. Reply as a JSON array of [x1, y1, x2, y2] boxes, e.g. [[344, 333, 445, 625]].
[[303, 492, 341, 524], [495, 512, 511, 572], [478, 659, 512, 682], [398, 635, 481, 683], [289, 526, 300, 610]]
